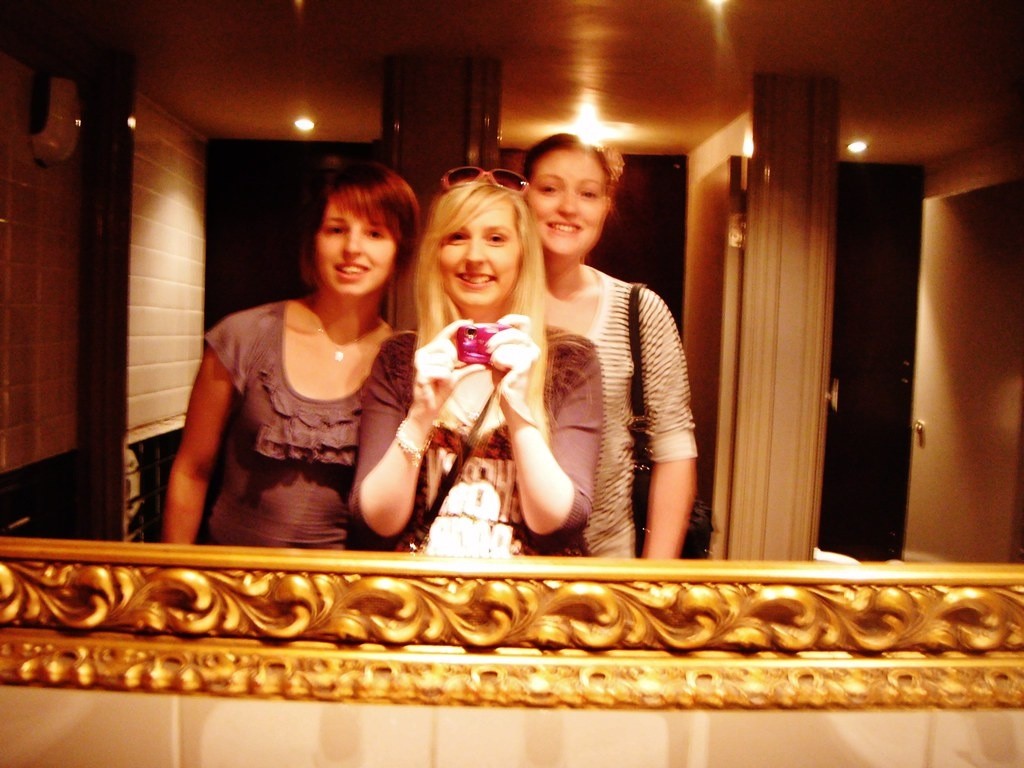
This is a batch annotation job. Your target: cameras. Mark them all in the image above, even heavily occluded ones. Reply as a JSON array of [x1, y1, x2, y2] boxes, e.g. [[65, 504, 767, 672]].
[[457, 323, 515, 365]]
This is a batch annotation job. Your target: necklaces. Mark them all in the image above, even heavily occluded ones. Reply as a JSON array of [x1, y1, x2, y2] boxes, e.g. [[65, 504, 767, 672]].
[[304, 305, 377, 342]]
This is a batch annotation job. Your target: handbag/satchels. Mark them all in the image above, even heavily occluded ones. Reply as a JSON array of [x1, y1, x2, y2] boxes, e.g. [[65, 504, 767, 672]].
[[633, 470, 712, 559]]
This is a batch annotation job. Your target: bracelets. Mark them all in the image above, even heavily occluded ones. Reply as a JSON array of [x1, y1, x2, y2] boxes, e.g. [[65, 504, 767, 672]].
[[395, 416, 434, 463]]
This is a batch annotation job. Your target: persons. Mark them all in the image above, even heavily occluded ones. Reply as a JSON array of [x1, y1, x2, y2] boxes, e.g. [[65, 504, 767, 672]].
[[349, 167, 604, 557], [161, 158, 421, 551], [514, 132, 700, 558]]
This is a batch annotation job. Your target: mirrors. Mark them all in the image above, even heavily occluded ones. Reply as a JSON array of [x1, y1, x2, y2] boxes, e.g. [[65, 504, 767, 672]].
[[0, 0, 1024, 709]]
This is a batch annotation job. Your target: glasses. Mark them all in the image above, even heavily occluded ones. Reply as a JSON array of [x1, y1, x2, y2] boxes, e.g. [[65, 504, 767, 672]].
[[440, 166, 530, 202]]
